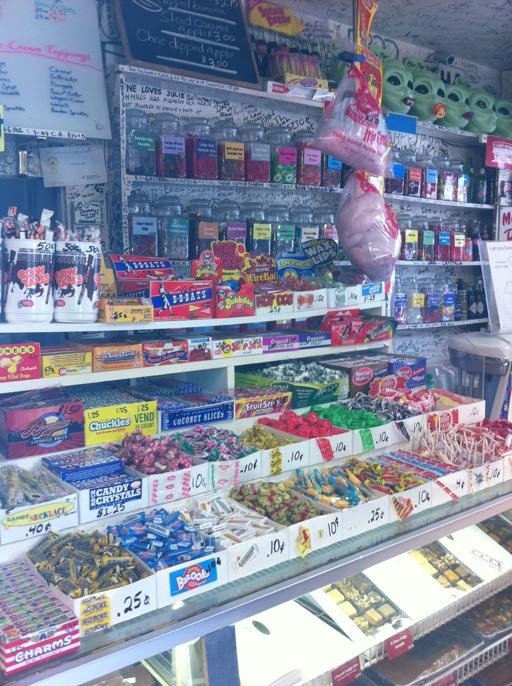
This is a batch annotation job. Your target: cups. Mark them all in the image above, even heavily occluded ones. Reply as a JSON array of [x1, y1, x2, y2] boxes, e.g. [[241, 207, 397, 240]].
[[3, 239, 101, 326]]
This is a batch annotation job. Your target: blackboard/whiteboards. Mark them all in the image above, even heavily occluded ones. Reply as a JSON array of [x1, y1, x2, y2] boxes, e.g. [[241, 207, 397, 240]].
[[116, 0, 263, 89]]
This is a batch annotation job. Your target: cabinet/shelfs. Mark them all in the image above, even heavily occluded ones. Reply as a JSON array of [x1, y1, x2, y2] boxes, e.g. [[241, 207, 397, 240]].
[[103, 62, 501, 339], [0, 238, 512, 686]]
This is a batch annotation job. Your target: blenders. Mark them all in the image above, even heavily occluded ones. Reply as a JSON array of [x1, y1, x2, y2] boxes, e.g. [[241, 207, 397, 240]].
[[447, 333, 511, 432]]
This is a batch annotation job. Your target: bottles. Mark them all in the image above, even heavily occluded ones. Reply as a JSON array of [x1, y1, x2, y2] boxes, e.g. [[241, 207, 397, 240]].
[[128, 201, 496, 260], [393, 273, 487, 329], [128, 109, 494, 201]]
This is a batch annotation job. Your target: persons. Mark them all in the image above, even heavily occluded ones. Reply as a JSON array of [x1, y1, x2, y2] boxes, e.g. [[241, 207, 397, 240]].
[[154, 282, 175, 315], [117, 254, 138, 279]]
